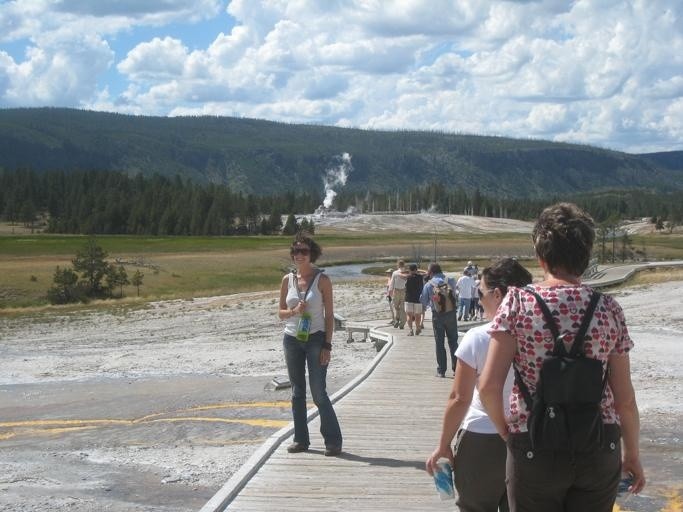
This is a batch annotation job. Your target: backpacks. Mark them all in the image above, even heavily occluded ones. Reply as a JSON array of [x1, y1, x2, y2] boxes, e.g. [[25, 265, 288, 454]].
[[428, 275, 454, 313], [512, 286, 609, 455]]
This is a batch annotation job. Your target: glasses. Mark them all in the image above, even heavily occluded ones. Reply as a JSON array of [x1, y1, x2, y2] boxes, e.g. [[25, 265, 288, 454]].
[[478, 288, 494, 297], [291, 248, 311, 256]]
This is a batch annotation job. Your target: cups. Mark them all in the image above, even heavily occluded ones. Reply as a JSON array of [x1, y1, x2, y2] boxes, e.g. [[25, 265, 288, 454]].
[[432, 460, 455, 500], [613, 469, 637, 506]]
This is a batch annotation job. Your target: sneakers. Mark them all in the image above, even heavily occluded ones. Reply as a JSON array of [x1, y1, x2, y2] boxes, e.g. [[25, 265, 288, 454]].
[[288, 442, 308, 452], [325, 443, 342, 456], [394, 321, 421, 336]]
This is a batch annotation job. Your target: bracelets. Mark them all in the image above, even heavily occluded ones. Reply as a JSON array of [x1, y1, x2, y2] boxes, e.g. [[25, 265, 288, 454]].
[[321, 342, 332, 351]]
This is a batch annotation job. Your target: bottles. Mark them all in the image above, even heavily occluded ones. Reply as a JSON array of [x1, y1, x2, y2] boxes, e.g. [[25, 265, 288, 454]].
[[296, 311, 312, 342]]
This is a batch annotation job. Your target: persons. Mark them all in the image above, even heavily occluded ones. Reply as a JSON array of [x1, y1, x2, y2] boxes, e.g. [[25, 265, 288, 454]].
[[279, 237, 342, 456], [426, 257, 533, 512], [385, 261, 485, 378], [479, 203, 646, 512]]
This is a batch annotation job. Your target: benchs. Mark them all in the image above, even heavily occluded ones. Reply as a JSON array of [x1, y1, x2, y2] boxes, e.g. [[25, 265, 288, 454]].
[[333, 313, 346, 333]]
